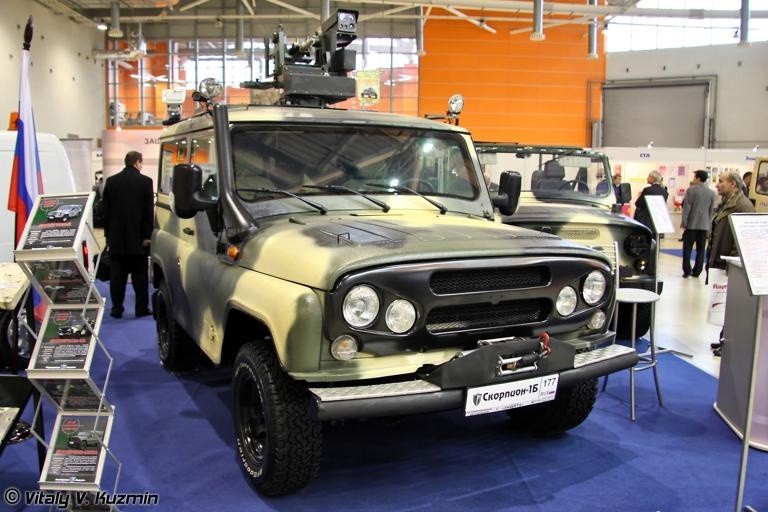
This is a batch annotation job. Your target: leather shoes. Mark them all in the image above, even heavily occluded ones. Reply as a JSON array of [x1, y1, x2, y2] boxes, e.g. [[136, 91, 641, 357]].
[[135, 307, 154, 318], [710, 338, 722, 356], [109, 305, 125, 318], [682, 270, 702, 278]]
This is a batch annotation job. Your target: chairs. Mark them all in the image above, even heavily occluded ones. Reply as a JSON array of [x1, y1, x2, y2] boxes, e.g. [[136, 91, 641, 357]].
[[231, 145, 278, 201], [533, 161, 573, 193], [599, 238, 666, 424]]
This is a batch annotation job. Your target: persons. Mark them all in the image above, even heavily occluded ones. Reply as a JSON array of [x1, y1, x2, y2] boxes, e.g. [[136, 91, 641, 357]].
[[679, 169, 715, 278], [101, 149, 155, 319], [595, 173, 623, 204], [758, 176, 768, 196], [705, 170, 757, 358], [741, 171, 756, 207], [633, 170, 668, 241], [677, 181, 696, 241]]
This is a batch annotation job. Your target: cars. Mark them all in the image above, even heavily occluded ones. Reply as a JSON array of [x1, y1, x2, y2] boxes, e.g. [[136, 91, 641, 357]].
[[48, 383, 88, 396], [56, 319, 95, 339]]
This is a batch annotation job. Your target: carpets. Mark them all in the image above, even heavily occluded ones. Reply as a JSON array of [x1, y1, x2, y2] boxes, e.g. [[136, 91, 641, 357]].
[[1, 279, 768, 510], [660, 249, 710, 266]]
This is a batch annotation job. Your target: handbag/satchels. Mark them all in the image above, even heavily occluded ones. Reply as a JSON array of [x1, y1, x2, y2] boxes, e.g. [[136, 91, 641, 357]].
[[94, 243, 113, 283], [706, 267, 729, 327]]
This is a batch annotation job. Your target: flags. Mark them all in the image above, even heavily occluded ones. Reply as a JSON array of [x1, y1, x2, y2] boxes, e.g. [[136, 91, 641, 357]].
[[8, 49, 52, 331]]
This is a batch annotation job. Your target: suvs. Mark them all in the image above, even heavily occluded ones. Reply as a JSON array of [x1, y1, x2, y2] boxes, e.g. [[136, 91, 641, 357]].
[[46, 204, 83, 222], [746, 154, 768, 213], [67, 431, 102, 450]]
